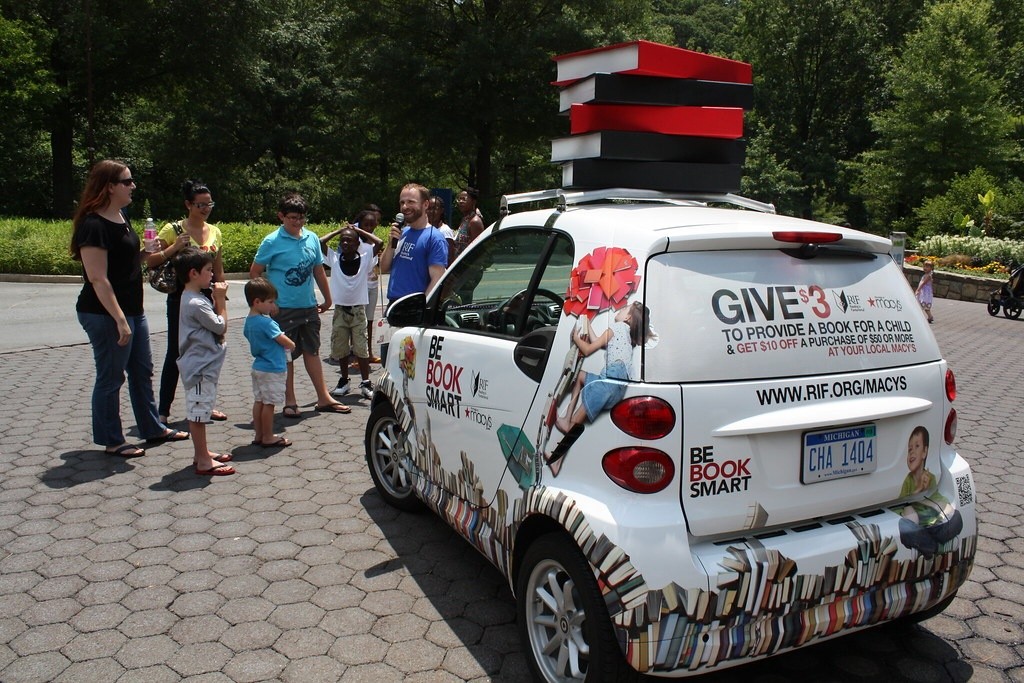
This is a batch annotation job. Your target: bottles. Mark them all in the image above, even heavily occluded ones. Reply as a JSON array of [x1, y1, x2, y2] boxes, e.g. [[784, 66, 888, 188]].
[[144, 218, 158, 252], [282, 331, 292, 362]]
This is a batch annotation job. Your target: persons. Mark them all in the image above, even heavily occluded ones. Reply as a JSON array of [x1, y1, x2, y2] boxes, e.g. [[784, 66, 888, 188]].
[[451, 187, 494, 307], [543, 300, 659, 477], [68, 160, 189, 458], [899, 425, 963, 561], [252, 199, 351, 417], [243, 276, 292, 448], [914, 261, 934, 323], [318, 223, 385, 400], [174, 246, 235, 476], [379, 184, 454, 369], [351, 203, 382, 363], [147, 179, 227, 426], [420, 408, 431, 447]]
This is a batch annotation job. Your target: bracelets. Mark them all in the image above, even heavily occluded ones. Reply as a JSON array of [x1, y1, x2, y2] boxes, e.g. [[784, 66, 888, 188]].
[[160, 249, 167, 261], [321, 287, 329, 293]]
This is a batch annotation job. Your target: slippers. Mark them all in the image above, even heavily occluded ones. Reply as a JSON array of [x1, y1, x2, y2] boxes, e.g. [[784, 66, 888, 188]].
[[195, 457, 236, 475], [251, 432, 291, 447], [209, 409, 227, 421], [283, 404, 301, 417], [315, 399, 352, 414], [104, 443, 145, 458], [145, 424, 190, 443], [192, 446, 233, 467]]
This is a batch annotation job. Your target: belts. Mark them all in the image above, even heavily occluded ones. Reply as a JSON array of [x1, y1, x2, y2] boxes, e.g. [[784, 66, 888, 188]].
[[340, 305, 361, 316]]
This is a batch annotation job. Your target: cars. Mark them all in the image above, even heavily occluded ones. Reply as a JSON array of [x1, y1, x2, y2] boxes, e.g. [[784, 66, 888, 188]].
[[364, 198, 979, 683]]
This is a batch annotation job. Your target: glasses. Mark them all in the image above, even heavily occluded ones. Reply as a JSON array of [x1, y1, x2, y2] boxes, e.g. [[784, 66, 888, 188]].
[[285, 215, 303, 222], [190, 202, 215, 209], [110, 178, 133, 187]]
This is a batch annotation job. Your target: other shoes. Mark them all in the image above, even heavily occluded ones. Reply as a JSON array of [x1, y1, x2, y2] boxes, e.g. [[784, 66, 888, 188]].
[[928, 316, 934, 321], [369, 354, 382, 362]]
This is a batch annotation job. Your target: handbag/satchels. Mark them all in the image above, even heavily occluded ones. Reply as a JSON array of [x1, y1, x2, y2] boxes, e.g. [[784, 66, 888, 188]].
[[148, 223, 190, 294]]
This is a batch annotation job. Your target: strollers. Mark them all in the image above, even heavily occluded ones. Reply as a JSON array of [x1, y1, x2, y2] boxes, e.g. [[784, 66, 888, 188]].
[[987, 264, 1024, 319]]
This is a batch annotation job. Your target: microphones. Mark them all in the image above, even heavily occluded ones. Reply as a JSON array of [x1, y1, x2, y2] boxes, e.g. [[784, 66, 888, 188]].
[[390, 213, 404, 249]]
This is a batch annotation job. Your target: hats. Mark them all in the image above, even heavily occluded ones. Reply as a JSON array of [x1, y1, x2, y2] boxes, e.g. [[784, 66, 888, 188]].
[[339, 250, 361, 276]]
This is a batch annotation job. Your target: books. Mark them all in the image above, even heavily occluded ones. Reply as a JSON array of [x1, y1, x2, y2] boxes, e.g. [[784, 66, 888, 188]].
[[377, 371, 411, 432], [399, 335, 416, 380], [405, 445, 508, 575], [842, 521, 976, 628], [549, 39, 755, 192], [648, 533, 849, 672], [503, 246, 642, 478], [513, 483, 649, 672]]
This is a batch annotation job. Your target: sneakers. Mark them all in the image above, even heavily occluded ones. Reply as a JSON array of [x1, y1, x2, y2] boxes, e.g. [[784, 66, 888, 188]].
[[358, 380, 374, 399], [332, 372, 350, 397]]
